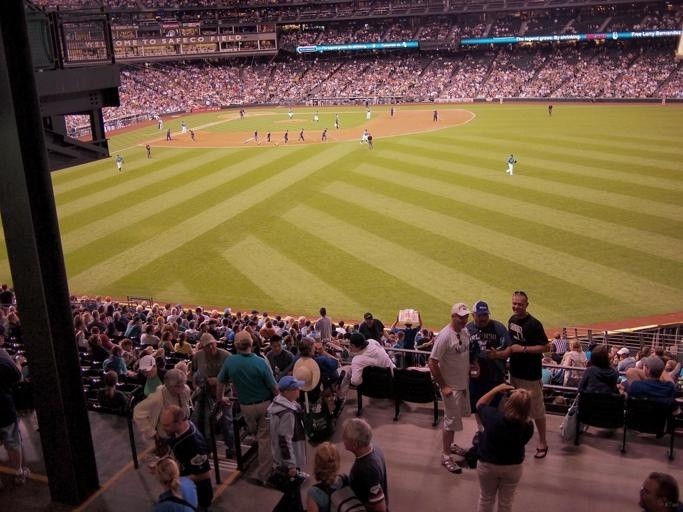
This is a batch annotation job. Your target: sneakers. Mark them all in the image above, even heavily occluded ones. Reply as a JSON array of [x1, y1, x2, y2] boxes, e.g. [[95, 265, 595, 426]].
[[16, 467, 30, 483]]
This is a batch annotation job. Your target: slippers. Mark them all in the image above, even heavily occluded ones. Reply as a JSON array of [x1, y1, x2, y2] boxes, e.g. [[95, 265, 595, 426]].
[[534, 445, 548, 458]]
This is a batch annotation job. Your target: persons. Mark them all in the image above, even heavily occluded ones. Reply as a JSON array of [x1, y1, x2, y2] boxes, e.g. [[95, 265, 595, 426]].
[[166, 127, 171, 141], [367, 132, 373, 150], [115, 154, 123, 171], [254, 130, 257, 141], [265, 132, 270, 142], [188, 129, 194, 142], [0, 280, 396, 512], [430, 290, 552, 474], [505, 153, 514, 175], [639, 472, 681, 512], [391, 311, 435, 368], [359, 129, 368, 143], [21, 1, 682, 135], [283, 129, 288, 143], [321, 131, 326, 141], [549, 333, 682, 425], [297, 128, 304, 141], [145, 144, 151, 159], [476, 383, 534, 512]]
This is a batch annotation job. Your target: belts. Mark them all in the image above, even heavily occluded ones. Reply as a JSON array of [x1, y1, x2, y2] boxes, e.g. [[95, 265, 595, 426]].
[[247, 399, 267, 405]]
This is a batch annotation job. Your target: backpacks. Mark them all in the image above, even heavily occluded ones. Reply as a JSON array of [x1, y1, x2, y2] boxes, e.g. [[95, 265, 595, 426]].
[[565, 351, 586, 380], [313, 473, 367, 512]]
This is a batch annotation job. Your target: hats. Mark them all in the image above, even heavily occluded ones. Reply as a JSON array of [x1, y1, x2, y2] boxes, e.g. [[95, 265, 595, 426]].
[[293, 357, 321, 392], [234, 330, 252, 343], [200, 333, 216, 347], [473, 300, 490, 315], [451, 303, 471, 317], [139, 356, 155, 372], [277, 375, 305, 389], [617, 347, 630, 355]]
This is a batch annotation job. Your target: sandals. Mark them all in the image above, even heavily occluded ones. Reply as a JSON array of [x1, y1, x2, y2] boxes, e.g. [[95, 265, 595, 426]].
[[441, 455, 461, 473], [451, 444, 465, 456]]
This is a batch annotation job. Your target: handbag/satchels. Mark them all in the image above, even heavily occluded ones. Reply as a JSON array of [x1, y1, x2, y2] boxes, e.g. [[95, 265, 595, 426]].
[[302, 395, 333, 447], [266, 467, 310, 489], [562, 393, 587, 440]]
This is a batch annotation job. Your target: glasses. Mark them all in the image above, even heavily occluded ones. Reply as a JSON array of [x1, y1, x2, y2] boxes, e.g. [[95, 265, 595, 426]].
[[457, 333, 462, 345]]
